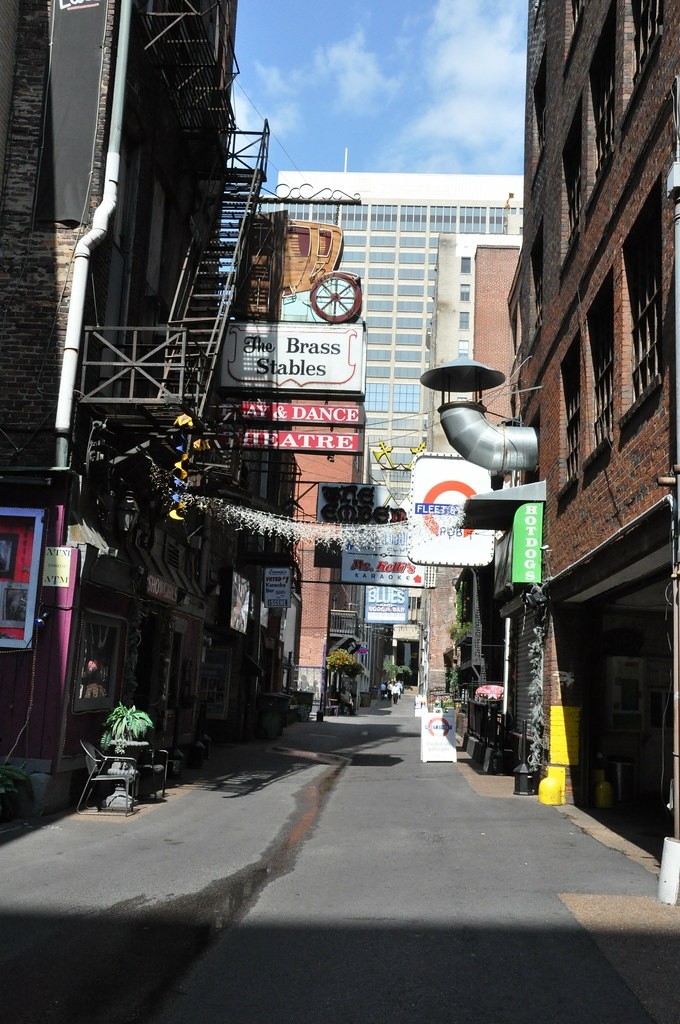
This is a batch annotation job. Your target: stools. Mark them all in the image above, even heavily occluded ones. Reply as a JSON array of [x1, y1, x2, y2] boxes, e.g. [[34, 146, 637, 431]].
[[611, 757, 635, 801]]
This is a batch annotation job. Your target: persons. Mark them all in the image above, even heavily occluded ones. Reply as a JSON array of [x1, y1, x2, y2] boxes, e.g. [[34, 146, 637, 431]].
[[380, 680, 404, 705], [340, 687, 353, 716]]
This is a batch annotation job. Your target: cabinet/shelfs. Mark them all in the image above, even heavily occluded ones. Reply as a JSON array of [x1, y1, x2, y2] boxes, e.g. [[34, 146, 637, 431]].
[[604, 655, 644, 798], [641, 658, 673, 797]]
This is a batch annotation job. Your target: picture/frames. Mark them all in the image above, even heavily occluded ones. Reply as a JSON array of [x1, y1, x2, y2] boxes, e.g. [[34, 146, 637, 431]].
[[199, 645, 231, 721], [70, 608, 129, 716], [1, 501, 49, 649]]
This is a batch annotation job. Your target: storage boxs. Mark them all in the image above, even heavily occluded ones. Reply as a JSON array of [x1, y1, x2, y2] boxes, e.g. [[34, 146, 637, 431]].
[[583, 733, 620, 770]]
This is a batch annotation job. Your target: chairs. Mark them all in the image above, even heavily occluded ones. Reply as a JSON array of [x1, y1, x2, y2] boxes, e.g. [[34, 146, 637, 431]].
[[140, 749, 168, 800], [77, 739, 139, 816]]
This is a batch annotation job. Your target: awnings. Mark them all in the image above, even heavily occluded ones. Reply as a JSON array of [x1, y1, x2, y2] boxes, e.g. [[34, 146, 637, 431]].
[[457, 480, 545, 529]]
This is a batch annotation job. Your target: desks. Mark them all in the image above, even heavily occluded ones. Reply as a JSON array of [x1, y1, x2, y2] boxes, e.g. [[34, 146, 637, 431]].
[[137, 768, 157, 803]]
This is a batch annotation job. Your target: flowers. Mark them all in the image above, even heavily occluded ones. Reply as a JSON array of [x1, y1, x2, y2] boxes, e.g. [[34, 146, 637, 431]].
[[476, 685, 504, 699], [326, 648, 354, 672]]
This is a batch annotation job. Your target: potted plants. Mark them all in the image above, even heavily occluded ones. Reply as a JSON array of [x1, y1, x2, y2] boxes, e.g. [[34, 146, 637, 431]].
[[101, 702, 153, 767], [448, 624, 460, 639], [0, 763, 34, 822]]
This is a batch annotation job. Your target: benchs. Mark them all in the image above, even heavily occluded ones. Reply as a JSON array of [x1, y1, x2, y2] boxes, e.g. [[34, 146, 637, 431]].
[[329, 698, 353, 717]]
